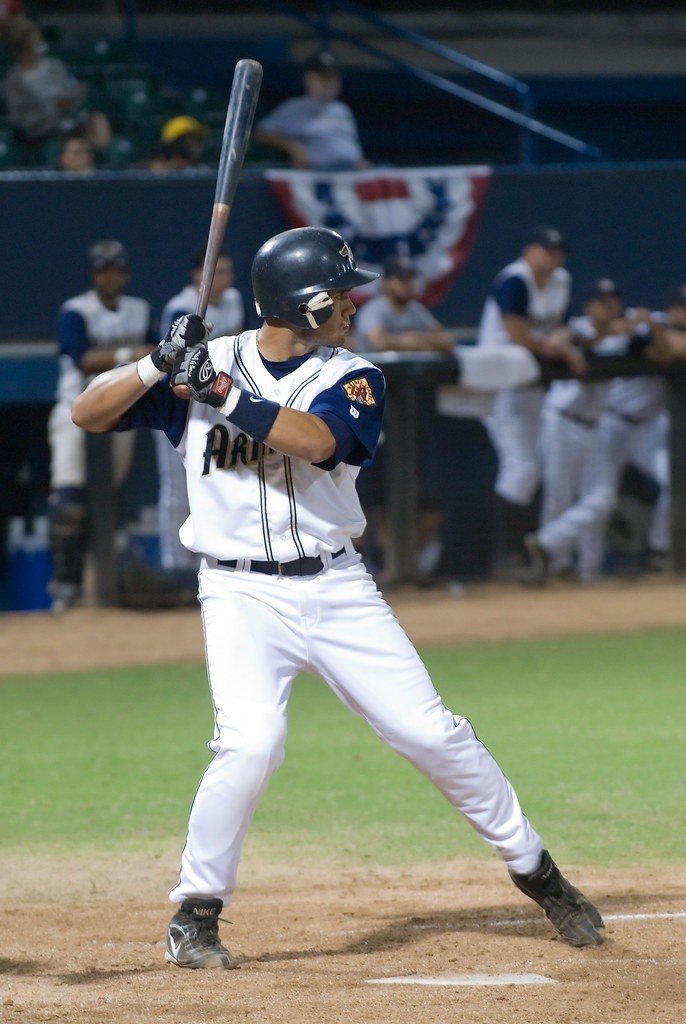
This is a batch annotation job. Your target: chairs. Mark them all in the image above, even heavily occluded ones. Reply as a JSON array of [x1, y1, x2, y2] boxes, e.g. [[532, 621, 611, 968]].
[[0, 25, 292, 172]]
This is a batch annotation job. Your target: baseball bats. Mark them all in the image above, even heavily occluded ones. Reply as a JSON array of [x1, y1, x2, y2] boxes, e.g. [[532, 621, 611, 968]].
[[173, 58, 264, 399]]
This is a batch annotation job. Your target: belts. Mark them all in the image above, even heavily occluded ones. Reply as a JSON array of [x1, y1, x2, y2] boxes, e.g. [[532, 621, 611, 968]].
[[552, 409, 600, 430], [218, 546, 343, 576], [607, 408, 653, 427]]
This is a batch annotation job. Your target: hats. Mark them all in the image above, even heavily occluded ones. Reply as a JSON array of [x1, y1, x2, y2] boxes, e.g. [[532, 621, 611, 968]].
[[582, 278, 619, 301], [520, 225, 570, 259], [161, 118, 210, 145], [385, 256, 423, 281], [304, 52, 342, 76]]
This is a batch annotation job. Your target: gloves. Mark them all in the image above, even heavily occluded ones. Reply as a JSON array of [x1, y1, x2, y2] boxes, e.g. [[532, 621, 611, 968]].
[[136, 312, 214, 387], [168, 342, 242, 419]]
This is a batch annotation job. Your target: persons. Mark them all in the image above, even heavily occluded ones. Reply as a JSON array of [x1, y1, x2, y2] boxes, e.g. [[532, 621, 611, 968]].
[[0, 19, 91, 143], [131, 117, 206, 169], [72, 227, 605, 968], [79, 116, 111, 150], [49, 241, 151, 613], [54, 136, 96, 174], [356, 260, 455, 574], [151, 251, 244, 572], [468, 225, 686, 580], [254, 51, 371, 171]]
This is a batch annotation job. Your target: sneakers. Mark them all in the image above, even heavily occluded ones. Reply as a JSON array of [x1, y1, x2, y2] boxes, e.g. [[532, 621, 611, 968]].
[[165, 897, 239, 970], [507, 850, 606, 947]]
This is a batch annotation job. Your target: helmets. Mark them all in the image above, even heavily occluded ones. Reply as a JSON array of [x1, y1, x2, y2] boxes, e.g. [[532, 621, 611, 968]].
[[251, 227, 380, 329]]
